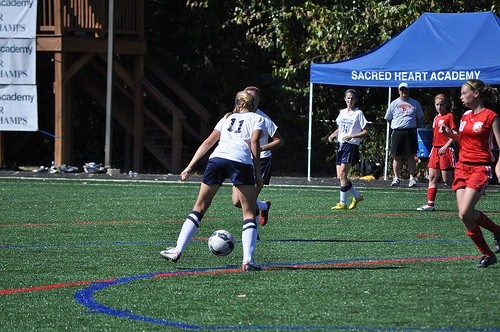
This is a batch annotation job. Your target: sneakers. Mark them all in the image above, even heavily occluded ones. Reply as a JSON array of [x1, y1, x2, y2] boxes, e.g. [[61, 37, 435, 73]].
[[417, 205, 435, 211], [49, 166, 58, 173], [160, 248, 181, 263], [33, 166, 48, 173], [493, 224, 500, 254], [409, 180, 416, 187], [259, 200, 271, 225], [331, 203, 347, 210], [391, 178, 400, 187], [348, 193, 364, 210], [476, 255, 497, 268], [242, 259, 261, 271]]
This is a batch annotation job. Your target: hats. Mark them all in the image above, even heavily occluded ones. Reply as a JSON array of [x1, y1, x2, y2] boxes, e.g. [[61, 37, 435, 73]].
[[399, 82, 408, 88]]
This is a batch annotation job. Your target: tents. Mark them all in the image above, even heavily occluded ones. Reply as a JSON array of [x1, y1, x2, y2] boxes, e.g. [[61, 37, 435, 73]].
[[306, 10, 500, 182]]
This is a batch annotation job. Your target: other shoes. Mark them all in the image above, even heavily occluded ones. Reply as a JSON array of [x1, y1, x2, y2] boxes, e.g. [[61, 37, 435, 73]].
[[59, 163, 109, 174]]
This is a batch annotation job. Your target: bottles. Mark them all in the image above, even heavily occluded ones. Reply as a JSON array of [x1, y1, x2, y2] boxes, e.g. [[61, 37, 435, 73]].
[[89, 162, 96, 166], [51, 161, 54, 168]]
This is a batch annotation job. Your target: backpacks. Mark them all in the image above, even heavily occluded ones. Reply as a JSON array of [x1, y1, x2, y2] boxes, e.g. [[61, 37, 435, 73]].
[[359, 159, 380, 179]]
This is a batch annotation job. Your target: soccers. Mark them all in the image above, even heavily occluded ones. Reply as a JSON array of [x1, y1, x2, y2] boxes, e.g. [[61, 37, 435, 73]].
[[208, 230, 236, 258]]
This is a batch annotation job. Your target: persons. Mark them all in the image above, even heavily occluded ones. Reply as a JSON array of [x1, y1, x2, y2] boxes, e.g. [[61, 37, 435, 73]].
[[328, 89, 369, 210], [416, 94, 458, 211], [160, 91, 265, 271], [231, 86, 285, 226], [384, 82, 425, 188], [438, 79, 500, 268]]
